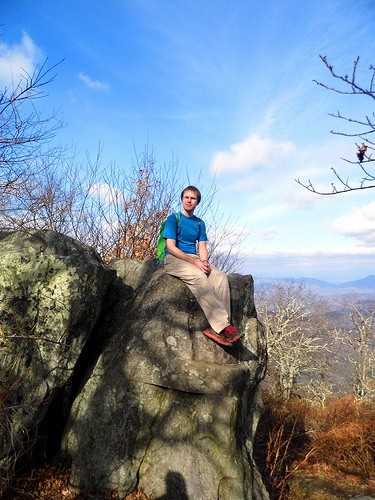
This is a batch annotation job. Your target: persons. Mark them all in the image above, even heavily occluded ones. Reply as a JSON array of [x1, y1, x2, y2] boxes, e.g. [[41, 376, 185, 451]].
[[162, 185, 245, 347]]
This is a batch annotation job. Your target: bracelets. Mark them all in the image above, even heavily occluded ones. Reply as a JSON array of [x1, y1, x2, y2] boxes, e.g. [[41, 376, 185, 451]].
[[201, 260, 209, 263]]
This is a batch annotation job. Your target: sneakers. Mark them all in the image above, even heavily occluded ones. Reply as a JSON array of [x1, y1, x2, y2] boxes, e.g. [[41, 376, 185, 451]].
[[203, 326, 233, 347], [221, 324, 245, 342]]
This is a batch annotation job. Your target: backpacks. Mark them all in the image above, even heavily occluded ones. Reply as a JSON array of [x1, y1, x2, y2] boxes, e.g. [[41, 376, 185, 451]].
[[157, 212, 200, 266]]
